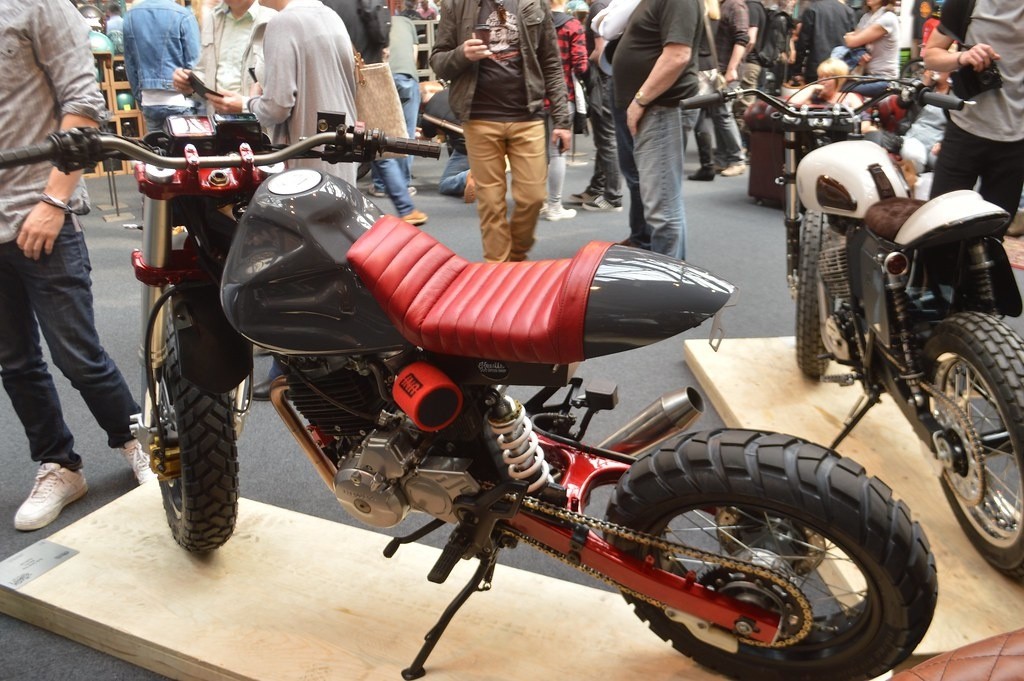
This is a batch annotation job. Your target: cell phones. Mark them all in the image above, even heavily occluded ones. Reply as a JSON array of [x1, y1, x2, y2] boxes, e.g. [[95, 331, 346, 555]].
[[475, 26, 490, 50]]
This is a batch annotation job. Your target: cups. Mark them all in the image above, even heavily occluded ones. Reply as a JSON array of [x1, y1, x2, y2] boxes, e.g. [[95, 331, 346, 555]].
[[475, 24, 491, 49]]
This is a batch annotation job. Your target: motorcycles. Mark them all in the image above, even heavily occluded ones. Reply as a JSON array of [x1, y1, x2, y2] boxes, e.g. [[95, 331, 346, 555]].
[[681, 82, 1024, 580], [0, 118, 938, 681]]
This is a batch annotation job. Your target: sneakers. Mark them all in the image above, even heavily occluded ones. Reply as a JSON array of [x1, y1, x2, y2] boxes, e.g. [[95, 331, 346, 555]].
[[119, 436, 158, 485], [14, 462, 88, 531]]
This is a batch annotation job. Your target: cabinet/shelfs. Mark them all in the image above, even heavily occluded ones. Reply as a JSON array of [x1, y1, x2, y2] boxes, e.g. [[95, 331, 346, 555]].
[[81, 55, 147, 177]]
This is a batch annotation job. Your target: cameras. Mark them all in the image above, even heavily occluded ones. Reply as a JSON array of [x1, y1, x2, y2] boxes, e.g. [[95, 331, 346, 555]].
[[968, 60, 1004, 93]]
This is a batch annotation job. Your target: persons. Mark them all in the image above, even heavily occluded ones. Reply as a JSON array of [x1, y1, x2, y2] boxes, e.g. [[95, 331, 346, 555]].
[[923, 0, 1024, 244], [0, 0, 157, 532], [123, 0, 202, 134], [246, 0, 358, 401], [322, 0, 1024, 262], [612, 0, 705, 261], [106, 4, 123, 35], [172, 0, 279, 142], [429, 0, 573, 263]]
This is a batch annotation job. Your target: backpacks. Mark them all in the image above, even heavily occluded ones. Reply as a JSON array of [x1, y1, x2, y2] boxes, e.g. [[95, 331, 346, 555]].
[[746, 1, 797, 67]]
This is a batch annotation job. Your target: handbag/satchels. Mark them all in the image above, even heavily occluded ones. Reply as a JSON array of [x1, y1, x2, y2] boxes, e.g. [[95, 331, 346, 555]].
[[349, 46, 412, 160], [714, 73, 727, 93]]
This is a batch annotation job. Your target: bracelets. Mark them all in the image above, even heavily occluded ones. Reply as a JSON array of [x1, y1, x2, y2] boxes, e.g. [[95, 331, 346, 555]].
[[957, 52, 963, 66], [40, 193, 72, 210], [184, 91, 196, 100], [635, 90, 648, 107]]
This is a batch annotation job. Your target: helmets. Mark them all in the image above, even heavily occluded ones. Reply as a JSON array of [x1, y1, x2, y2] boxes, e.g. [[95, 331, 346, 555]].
[[566, 1, 590, 22]]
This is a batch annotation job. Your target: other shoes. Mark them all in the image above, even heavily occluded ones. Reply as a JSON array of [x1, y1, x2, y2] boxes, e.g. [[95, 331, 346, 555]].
[[687, 164, 715, 180], [546, 204, 577, 221], [400, 209, 428, 225], [582, 195, 623, 212], [1007, 211, 1024, 235], [711, 147, 725, 155], [245, 376, 276, 401], [710, 163, 727, 174], [569, 190, 601, 203], [463, 169, 477, 202], [367, 183, 386, 197], [721, 163, 746, 176], [407, 186, 418, 197], [538, 201, 549, 216]]
